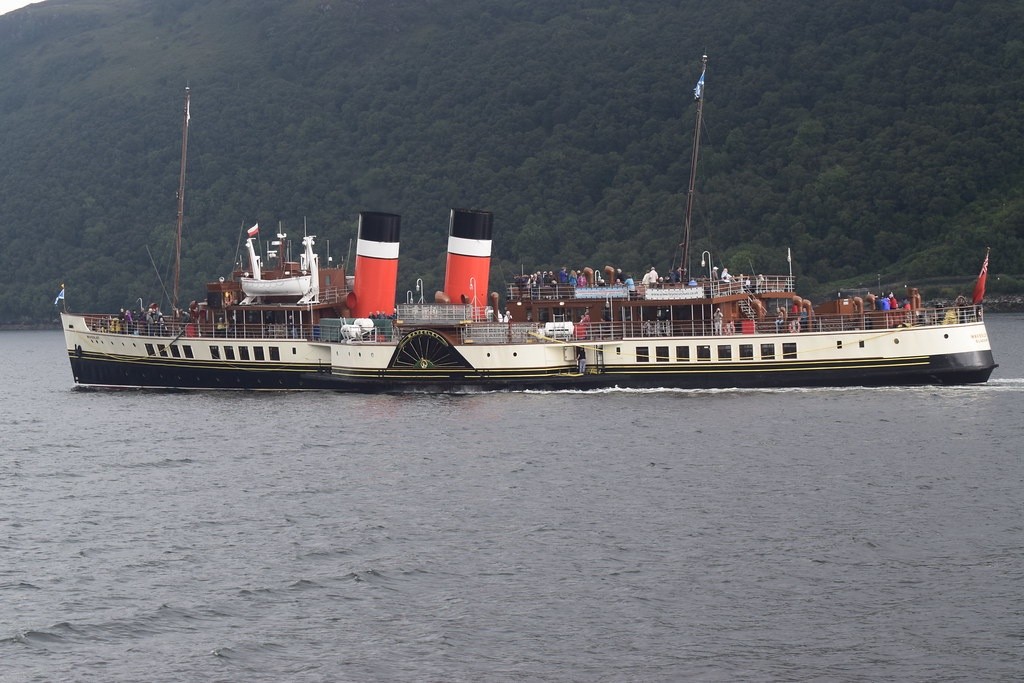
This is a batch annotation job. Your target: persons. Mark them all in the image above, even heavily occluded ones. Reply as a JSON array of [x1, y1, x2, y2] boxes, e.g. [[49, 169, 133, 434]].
[[711, 267, 720, 281], [776, 307, 787, 332], [668, 270, 675, 283], [580, 312, 591, 340], [722, 268, 731, 284], [513, 267, 587, 299], [642, 270, 650, 288], [504, 311, 512, 322], [613, 269, 636, 296], [118, 308, 168, 336], [800, 307, 818, 332], [649, 267, 658, 288], [675, 267, 682, 283], [755, 274, 764, 292], [714, 308, 722, 335], [368, 309, 397, 319], [875, 292, 911, 328]]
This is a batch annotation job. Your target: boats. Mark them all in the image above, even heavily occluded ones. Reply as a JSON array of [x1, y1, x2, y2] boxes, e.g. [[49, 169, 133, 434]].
[[54, 55, 1000, 393]]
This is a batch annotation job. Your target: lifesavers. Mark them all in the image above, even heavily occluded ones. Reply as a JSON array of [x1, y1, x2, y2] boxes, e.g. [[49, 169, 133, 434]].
[[724, 323, 735, 335], [224, 293, 233, 305], [788, 322, 801, 332]]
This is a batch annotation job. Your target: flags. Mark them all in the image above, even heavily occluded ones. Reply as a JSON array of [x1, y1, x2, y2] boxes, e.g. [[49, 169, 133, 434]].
[[55, 289, 64, 304], [247, 223, 259, 236], [973, 257, 988, 303], [693, 70, 706, 99]]
